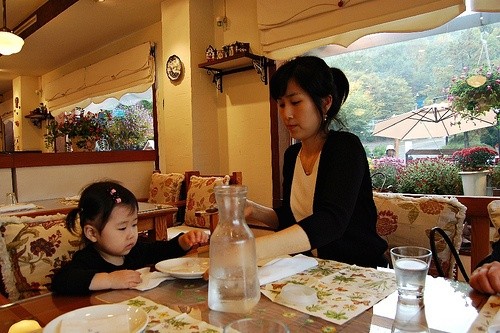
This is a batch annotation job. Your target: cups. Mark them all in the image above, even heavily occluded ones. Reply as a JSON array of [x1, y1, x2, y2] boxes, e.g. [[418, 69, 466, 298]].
[[5, 192, 16, 206], [223, 316, 290, 333]]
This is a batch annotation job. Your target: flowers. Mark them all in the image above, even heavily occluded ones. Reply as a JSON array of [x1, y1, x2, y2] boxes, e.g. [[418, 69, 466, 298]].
[[52, 108, 107, 141], [453, 146, 497, 171], [448, 66, 500, 129]]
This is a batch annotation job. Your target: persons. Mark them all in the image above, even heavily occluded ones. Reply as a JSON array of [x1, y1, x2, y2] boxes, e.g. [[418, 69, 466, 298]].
[[469, 229, 500, 294], [51, 181, 208, 295], [386, 144, 396, 158], [202, 55, 390, 281]]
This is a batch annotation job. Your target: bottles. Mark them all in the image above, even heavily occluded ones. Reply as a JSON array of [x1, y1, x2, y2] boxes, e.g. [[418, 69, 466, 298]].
[[390, 246, 432, 333], [207, 186, 264, 314]]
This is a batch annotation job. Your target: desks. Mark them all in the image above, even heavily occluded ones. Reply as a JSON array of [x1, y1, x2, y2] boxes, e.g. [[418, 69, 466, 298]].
[[1, 244, 500, 333], [0, 194, 178, 305]]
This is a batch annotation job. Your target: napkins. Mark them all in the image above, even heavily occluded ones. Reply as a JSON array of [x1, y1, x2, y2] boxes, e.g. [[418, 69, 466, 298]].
[[64, 195, 82, 202], [258, 252, 318, 286], [128, 267, 173, 293], [0, 202, 36, 214]]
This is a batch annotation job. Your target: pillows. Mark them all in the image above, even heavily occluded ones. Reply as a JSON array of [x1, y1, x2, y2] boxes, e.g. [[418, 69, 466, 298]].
[[149, 172, 183, 224], [183, 174, 231, 228], [0, 212, 85, 302], [369, 192, 466, 280]]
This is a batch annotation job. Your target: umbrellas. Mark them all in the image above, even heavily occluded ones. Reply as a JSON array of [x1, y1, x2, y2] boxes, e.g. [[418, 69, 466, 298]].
[[370, 103, 499, 143]]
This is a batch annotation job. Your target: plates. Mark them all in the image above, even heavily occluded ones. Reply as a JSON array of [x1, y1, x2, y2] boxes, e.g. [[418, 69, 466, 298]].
[[165, 55, 182, 80], [43, 303, 149, 333], [155, 256, 210, 279]]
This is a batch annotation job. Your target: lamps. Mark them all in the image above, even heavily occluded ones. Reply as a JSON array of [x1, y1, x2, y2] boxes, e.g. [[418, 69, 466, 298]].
[[0, 0, 24, 56]]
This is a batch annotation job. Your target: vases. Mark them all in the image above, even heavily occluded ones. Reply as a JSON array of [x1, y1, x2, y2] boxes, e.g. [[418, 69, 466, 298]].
[[456, 169, 489, 197], [461, 85, 500, 115], [71, 131, 100, 152]]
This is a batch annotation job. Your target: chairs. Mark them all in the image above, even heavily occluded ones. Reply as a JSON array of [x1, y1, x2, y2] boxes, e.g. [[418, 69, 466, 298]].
[[147, 170, 188, 226], [167, 169, 242, 242]]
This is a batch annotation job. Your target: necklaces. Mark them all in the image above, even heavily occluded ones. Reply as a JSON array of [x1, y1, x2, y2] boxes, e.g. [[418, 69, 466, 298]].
[[301, 146, 320, 175]]
